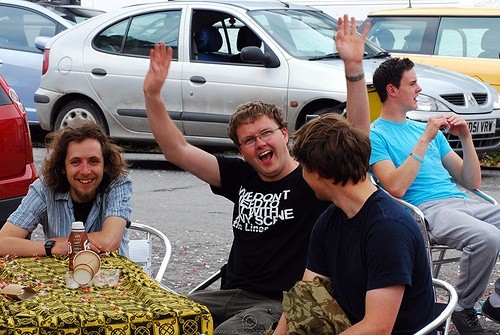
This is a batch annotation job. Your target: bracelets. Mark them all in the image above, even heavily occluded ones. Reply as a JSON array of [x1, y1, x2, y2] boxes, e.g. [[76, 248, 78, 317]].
[[409, 152, 425, 164], [345, 73, 365, 81]]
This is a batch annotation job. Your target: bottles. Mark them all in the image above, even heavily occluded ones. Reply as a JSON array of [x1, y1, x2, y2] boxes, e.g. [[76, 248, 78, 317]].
[[68, 221, 89, 271]]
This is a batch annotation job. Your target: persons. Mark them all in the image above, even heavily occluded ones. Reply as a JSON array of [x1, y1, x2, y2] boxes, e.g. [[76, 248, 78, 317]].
[[273, 113, 438, 335], [142, 14, 371, 335], [0, 123, 133, 260], [368, 58, 500, 335]]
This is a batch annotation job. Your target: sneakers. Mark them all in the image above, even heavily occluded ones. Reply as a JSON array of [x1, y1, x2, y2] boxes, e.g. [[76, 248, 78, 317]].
[[452, 307, 486, 335], [481, 296, 500, 322]]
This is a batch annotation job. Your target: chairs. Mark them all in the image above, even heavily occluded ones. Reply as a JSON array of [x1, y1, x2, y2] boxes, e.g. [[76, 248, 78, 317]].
[[370, 176, 500, 279], [377, 26, 424, 52], [478, 30, 500, 59], [128, 221, 171, 289], [412, 277, 459, 335], [8, 18, 68, 50], [194, 22, 268, 63]]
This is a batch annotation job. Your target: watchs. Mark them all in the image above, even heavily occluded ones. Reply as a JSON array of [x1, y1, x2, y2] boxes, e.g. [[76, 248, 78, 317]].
[[44, 240, 56, 258]]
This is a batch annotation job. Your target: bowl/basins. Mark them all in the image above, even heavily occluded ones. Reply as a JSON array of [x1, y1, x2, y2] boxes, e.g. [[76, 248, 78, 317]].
[[66, 269, 119, 289]]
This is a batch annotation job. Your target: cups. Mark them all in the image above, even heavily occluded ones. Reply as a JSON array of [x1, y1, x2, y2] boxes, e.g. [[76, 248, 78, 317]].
[[72, 250, 101, 285]]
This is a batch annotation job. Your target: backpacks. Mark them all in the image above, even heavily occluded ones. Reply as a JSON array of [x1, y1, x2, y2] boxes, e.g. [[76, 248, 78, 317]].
[[281, 278, 353, 335]]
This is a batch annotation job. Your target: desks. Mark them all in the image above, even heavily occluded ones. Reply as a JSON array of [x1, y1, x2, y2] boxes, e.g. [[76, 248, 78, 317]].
[[0, 252, 214, 335]]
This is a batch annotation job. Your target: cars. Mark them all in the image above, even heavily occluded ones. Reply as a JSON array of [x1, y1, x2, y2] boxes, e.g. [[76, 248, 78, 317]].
[[33, 0, 500, 147], [0, 1, 150, 126], [0, 73, 40, 223], [344, 5, 500, 97]]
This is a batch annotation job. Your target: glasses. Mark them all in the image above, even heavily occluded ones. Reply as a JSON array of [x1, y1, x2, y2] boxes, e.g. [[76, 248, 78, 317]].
[[237, 125, 282, 146]]
